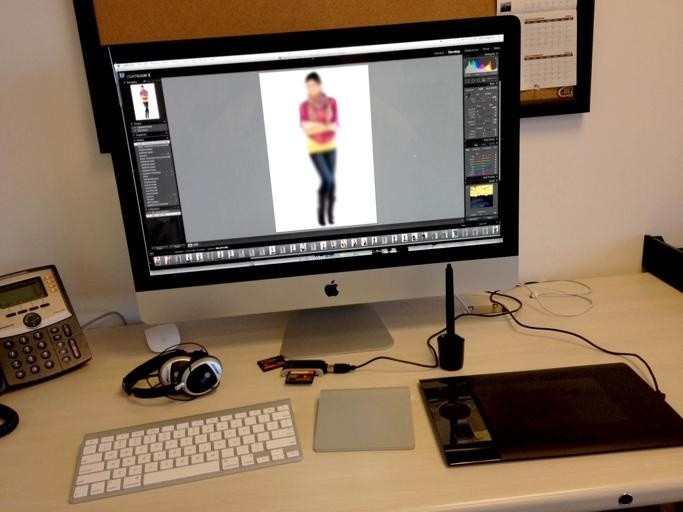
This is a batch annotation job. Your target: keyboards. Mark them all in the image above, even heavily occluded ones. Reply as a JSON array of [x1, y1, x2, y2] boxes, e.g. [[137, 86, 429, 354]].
[[68, 399, 304, 503]]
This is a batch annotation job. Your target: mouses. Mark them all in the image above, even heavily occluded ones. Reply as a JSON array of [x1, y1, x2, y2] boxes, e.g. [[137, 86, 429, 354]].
[[144, 323, 181, 353]]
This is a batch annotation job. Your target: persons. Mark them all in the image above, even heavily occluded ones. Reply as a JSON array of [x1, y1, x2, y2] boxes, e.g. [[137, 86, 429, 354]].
[[298, 73, 340, 225], [140, 86, 149, 118]]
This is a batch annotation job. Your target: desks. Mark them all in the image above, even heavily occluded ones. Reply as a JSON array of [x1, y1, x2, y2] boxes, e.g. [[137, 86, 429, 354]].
[[1, 270, 683, 511]]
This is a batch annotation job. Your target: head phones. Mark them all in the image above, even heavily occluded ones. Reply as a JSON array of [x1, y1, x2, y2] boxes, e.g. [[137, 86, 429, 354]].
[[122, 350, 223, 397]]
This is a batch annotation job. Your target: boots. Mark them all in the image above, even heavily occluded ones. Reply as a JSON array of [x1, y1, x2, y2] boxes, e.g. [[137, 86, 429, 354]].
[[327, 182, 336, 224], [317, 186, 326, 226]]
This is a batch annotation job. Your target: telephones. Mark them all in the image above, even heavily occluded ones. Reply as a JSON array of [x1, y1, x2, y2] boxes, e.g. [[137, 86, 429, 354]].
[[0, 264, 93, 395]]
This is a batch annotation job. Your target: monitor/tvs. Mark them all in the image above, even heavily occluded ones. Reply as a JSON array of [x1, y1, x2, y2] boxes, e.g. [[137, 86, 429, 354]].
[[91, 16, 521, 359]]
